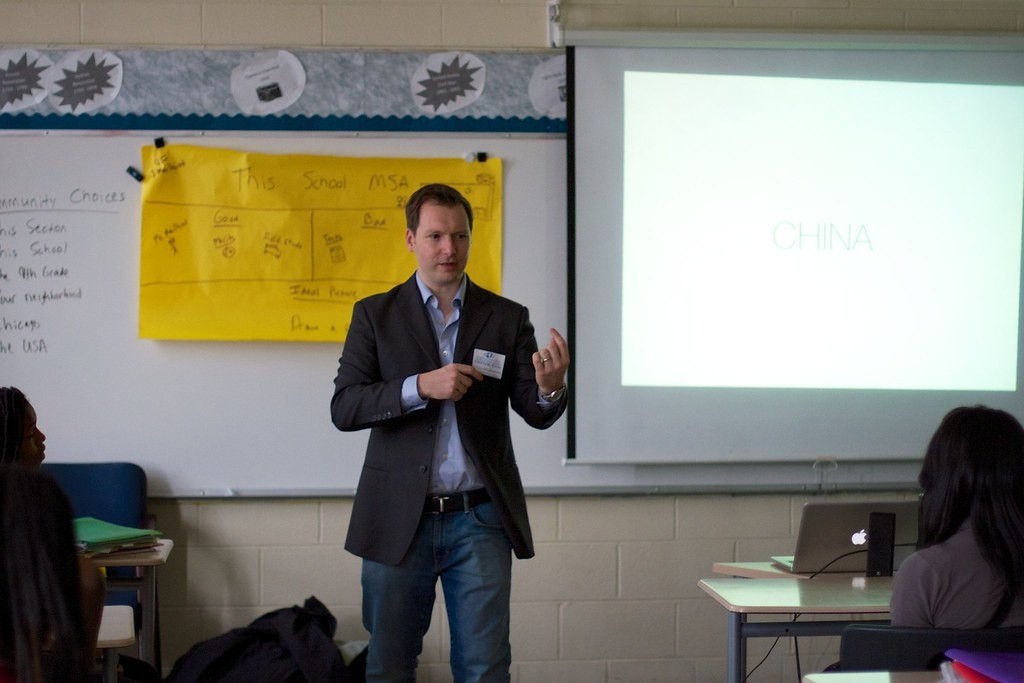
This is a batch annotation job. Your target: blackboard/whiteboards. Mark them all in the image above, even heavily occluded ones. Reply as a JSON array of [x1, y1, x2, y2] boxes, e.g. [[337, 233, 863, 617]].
[[0, 129, 1024, 499]]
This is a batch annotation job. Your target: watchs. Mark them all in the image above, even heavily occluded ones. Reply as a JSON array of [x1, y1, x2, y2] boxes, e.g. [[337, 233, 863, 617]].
[[544, 387, 565, 401]]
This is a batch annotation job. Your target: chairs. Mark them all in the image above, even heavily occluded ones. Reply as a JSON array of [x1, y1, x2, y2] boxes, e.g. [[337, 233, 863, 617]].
[[44, 460, 151, 634]]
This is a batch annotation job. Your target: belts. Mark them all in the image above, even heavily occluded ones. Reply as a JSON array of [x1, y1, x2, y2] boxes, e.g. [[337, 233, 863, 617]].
[[424, 487, 490, 516]]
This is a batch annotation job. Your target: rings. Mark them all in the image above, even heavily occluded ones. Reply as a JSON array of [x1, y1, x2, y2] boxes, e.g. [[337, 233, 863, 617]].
[[543, 357, 552, 362]]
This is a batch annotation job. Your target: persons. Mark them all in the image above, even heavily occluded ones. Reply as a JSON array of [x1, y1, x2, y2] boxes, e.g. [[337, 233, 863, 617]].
[[0, 387, 106, 683], [822, 406, 1024, 672], [330, 184, 570, 683]]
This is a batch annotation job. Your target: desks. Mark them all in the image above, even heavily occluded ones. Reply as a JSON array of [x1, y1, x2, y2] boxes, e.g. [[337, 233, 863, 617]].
[[90, 538, 175, 673], [713, 561, 898, 683], [97, 604, 136, 683], [803, 671, 944, 683], [697, 578, 892, 683]]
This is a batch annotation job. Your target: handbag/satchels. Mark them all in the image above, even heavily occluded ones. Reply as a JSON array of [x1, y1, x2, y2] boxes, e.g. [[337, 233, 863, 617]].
[[159, 596, 352, 683]]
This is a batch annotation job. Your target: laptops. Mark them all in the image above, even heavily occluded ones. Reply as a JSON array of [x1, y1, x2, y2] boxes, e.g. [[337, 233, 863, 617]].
[[771, 500, 920, 574]]
